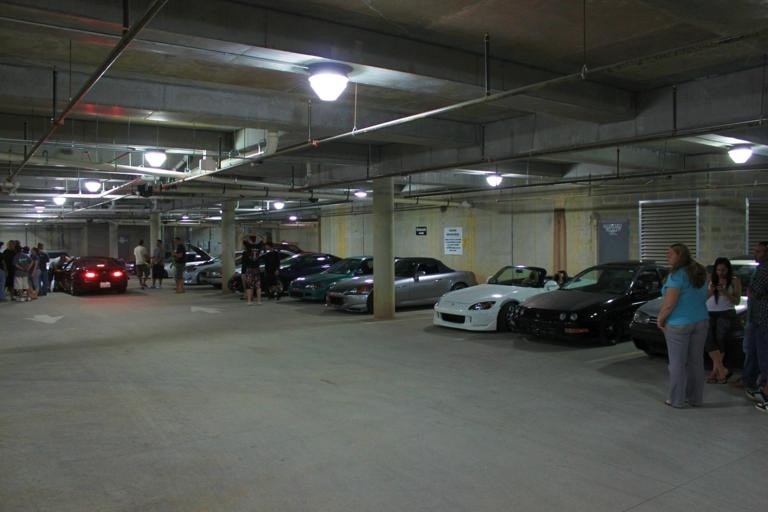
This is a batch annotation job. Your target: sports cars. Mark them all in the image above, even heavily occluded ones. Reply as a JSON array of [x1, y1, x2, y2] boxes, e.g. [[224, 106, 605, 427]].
[[64, 256, 128, 295], [289, 256, 401, 301], [326, 256, 476, 313], [432, 264, 597, 333]]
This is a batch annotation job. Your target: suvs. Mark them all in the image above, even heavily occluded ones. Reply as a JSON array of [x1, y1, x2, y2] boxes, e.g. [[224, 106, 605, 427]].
[[631, 258, 756, 360]]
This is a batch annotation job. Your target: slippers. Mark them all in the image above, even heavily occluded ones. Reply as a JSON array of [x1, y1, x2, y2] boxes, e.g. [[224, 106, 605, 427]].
[[707, 377, 718, 384], [719, 371, 734, 385]]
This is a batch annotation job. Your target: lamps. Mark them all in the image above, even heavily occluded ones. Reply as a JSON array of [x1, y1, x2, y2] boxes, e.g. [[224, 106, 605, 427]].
[[306, 62, 353, 102], [83, 179, 101, 193], [51, 192, 66, 206], [33, 205, 45, 213], [274, 201, 284, 210], [351, 188, 368, 198], [145, 148, 166, 168], [486, 173, 503, 188]]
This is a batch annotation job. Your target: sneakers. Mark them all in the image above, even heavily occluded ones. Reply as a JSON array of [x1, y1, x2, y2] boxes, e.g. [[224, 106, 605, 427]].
[[753, 401, 768, 413], [745, 387, 765, 402]]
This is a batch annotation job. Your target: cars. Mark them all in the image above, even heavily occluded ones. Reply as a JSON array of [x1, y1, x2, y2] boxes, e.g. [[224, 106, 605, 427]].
[[199, 250, 300, 289], [162, 252, 201, 279], [182, 244, 223, 285], [127, 261, 135, 272], [516, 260, 667, 346], [197, 251, 244, 288]]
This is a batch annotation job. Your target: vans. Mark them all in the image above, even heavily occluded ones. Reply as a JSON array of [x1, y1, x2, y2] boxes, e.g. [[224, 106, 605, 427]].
[[42, 250, 73, 270]]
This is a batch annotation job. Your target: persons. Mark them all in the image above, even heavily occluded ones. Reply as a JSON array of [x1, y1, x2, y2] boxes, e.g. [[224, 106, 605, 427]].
[[729, 241, 768, 388], [261, 240, 282, 300], [171, 237, 186, 293], [0, 238, 49, 302], [47, 253, 66, 292], [745, 387, 768, 413], [656, 244, 708, 409], [239, 233, 264, 306], [151, 239, 165, 289], [703, 257, 742, 385], [133, 239, 154, 290]]
